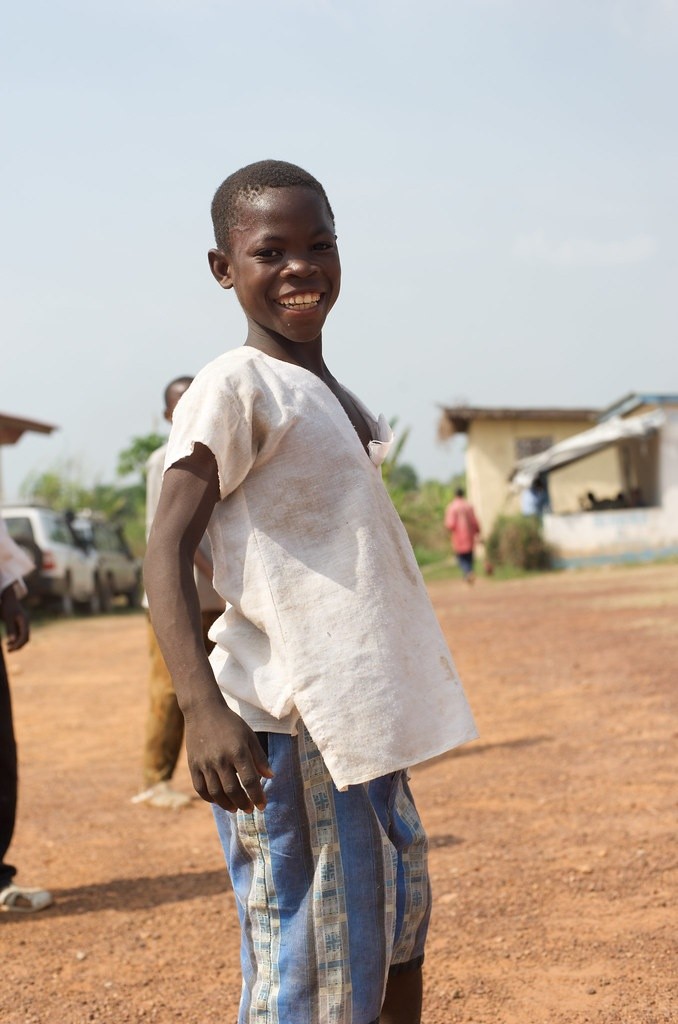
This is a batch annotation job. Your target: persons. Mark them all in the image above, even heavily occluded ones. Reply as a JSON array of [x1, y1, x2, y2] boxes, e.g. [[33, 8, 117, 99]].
[[144, 160, 486, 1024], [131, 377, 225, 809], [444, 488, 481, 581], [587, 491, 644, 512], [0, 517, 53, 913], [520, 480, 548, 517]]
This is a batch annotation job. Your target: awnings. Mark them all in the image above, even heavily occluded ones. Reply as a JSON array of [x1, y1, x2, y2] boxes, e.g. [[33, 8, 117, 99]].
[[506, 409, 666, 487]]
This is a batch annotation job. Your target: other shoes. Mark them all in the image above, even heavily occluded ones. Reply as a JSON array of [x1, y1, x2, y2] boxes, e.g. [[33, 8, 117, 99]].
[[131, 783, 191, 808], [0, 882, 54, 912]]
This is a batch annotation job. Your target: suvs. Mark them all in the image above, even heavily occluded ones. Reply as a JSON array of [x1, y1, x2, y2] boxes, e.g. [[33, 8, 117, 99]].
[[0, 504, 145, 618]]
